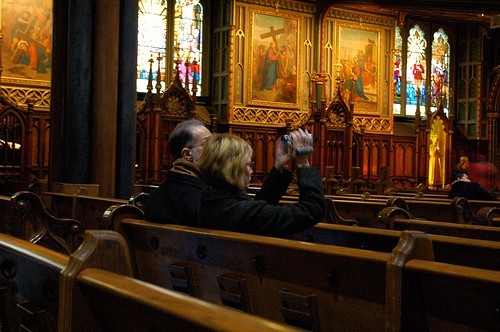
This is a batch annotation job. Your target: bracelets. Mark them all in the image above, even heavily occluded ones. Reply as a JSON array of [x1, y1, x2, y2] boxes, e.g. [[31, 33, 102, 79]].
[[298, 163, 309, 168]]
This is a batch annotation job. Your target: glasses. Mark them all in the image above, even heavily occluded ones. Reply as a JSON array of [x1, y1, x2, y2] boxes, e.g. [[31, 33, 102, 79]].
[[245, 162, 255, 168]]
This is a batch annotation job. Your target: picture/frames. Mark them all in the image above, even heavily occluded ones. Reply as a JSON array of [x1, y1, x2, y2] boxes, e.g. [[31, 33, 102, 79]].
[[0, 0, 53, 88], [331, 19, 385, 116], [245, 4, 305, 110]]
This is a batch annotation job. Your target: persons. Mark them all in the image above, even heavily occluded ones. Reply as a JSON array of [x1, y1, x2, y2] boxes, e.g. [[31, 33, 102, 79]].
[[448, 172, 497, 199], [145, 118, 213, 229], [196, 128, 327, 238]]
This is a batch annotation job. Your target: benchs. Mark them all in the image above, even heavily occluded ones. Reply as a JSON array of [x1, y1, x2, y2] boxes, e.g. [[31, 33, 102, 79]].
[[0, 184, 500, 332]]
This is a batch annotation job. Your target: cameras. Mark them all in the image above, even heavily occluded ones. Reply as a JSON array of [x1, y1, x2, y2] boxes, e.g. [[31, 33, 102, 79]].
[[281, 134, 313, 152]]
